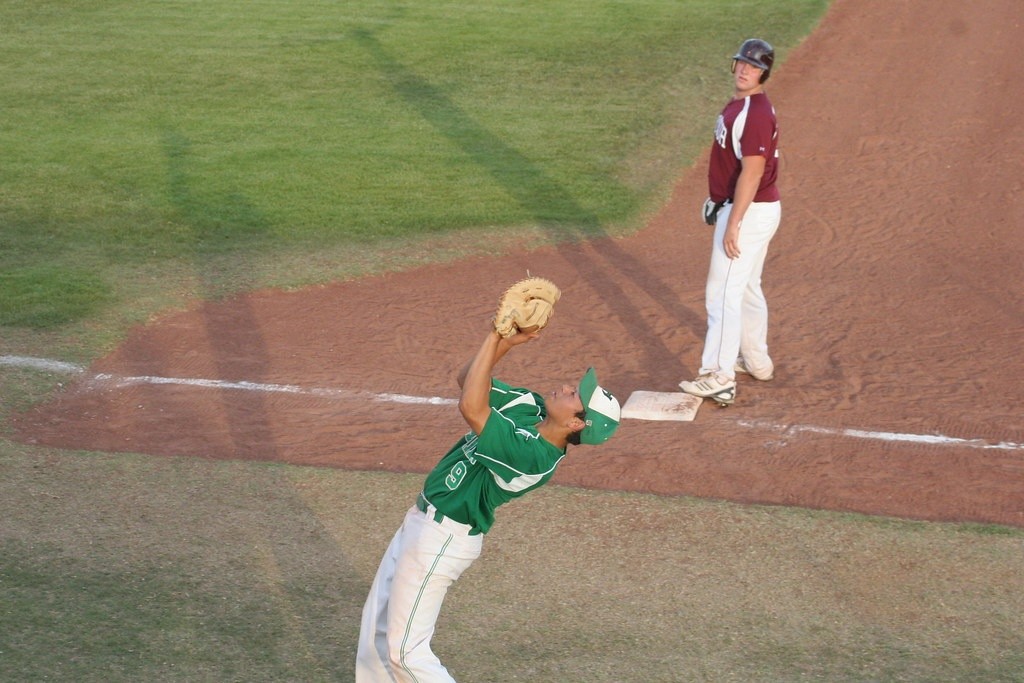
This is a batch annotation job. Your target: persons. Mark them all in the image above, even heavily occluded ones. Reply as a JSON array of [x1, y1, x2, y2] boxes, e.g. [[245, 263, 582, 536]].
[[355, 328, 620, 683], [679, 38, 781, 404]]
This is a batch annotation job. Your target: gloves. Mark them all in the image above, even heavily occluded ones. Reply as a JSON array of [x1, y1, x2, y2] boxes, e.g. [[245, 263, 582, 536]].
[[701, 195, 721, 227]]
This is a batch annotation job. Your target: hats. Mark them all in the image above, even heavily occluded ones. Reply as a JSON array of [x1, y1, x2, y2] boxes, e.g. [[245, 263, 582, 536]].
[[579, 367, 621, 445]]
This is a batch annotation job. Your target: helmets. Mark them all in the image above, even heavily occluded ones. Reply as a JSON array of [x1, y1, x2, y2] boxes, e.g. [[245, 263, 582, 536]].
[[730, 37, 774, 85]]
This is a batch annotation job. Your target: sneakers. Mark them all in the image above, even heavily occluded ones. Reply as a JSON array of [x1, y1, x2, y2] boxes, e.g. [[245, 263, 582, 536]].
[[679, 371, 736, 408], [737, 357, 774, 381]]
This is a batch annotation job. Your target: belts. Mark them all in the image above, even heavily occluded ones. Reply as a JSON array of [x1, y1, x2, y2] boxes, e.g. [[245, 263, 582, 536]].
[[416, 494, 480, 536]]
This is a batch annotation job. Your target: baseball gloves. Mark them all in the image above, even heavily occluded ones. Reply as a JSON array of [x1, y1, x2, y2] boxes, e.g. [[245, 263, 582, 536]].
[[492, 278, 562, 339]]
